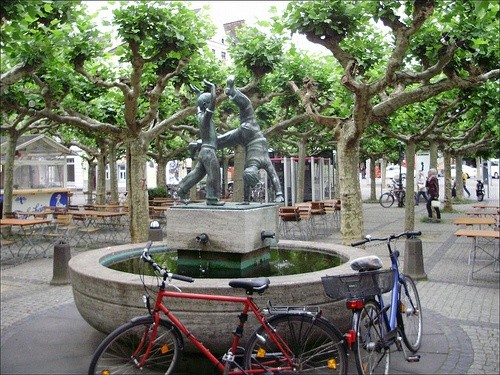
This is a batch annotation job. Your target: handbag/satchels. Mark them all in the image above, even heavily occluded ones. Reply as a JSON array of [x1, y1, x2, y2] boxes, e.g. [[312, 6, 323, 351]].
[[432, 199, 441, 208]]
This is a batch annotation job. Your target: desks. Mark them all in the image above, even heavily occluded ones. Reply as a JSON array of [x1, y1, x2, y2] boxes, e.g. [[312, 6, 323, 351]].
[[95, 205, 123, 211], [154, 206, 169, 217], [19, 212, 54, 219], [0, 219, 51, 264], [452, 204, 500, 285], [82, 211, 128, 244]]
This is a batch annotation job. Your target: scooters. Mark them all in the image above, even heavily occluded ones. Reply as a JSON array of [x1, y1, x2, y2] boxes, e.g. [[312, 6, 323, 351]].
[[476, 180, 485, 202]]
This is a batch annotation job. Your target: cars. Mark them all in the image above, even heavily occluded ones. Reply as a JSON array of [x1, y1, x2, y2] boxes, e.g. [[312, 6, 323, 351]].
[[386, 165, 416, 179], [437, 164, 478, 180], [480, 159, 499, 178]]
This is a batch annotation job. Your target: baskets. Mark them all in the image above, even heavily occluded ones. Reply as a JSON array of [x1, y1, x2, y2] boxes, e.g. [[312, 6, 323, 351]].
[[321, 268, 395, 300]]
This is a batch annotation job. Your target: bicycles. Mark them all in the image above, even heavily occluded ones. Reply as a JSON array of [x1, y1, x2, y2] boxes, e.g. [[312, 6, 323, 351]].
[[379, 177, 417, 208], [321, 231, 423, 375], [87, 240, 351, 375]]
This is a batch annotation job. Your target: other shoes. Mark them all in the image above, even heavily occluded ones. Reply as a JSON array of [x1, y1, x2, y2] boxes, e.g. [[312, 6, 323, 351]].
[[436, 219, 442, 224], [425, 217, 433, 223]]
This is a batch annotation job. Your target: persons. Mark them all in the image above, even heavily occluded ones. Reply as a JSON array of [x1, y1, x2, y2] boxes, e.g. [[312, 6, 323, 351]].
[[462, 169, 471, 198], [172, 78, 226, 206], [188, 75, 284, 205], [426, 168, 441, 223], [414, 170, 429, 205]]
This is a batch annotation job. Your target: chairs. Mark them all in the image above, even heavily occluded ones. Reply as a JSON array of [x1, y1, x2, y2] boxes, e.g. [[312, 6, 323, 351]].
[[278, 199, 342, 242], [0, 200, 167, 271]]
